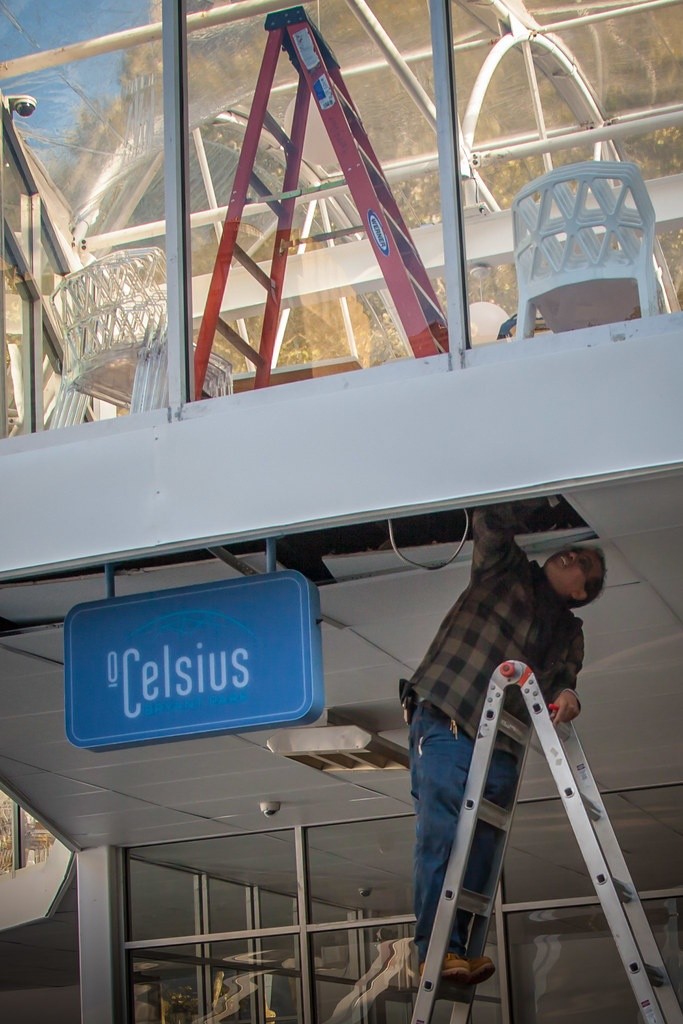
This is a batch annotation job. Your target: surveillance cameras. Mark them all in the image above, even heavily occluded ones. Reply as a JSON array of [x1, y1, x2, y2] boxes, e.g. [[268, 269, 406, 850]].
[[359, 888, 371, 897], [260, 801, 280, 816], [14, 98, 36, 117]]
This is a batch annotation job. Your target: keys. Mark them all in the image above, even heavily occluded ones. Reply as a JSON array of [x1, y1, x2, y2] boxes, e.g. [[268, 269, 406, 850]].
[[450, 717, 459, 740]]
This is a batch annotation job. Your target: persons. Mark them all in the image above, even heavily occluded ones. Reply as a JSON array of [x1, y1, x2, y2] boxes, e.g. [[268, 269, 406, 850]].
[[397, 504, 607, 986]]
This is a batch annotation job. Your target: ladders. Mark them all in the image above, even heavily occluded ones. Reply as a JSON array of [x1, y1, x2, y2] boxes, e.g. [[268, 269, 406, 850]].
[[190, 7, 451, 398], [411, 658, 682, 1024]]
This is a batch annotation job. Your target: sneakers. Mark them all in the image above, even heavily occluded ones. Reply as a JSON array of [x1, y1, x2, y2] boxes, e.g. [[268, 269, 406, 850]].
[[419, 952, 469, 982], [467, 957, 495, 988]]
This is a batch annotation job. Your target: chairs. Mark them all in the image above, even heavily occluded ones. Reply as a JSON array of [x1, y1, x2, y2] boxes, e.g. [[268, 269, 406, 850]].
[[40, 246, 235, 427], [511, 156, 678, 343]]
